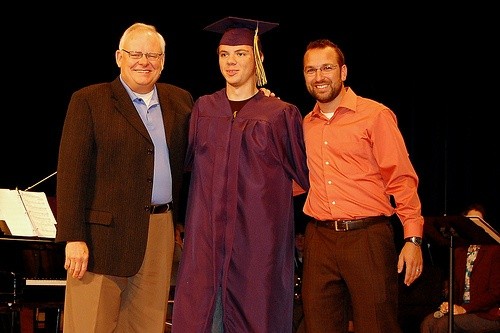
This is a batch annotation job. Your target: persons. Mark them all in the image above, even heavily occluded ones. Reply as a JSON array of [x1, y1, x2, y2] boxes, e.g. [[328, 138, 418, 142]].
[[171, 17, 310, 333], [55, 23, 275, 333], [421, 207, 500, 333], [292, 39, 425, 333]]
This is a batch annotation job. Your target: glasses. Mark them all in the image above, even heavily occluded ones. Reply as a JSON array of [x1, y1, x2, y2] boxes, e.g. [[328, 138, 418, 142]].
[[122, 49, 163, 61], [303, 65, 340, 74]]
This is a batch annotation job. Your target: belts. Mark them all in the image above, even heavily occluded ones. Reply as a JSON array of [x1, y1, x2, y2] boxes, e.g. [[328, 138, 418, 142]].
[[150, 201, 174, 215], [311, 215, 383, 232]]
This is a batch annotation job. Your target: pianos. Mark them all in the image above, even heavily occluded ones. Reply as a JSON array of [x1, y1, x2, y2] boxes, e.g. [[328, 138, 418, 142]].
[[0, 188, 68, 333]]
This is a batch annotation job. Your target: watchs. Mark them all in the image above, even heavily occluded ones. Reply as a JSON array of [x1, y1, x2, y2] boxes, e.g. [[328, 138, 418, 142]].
[[405, 236, 422, 246]]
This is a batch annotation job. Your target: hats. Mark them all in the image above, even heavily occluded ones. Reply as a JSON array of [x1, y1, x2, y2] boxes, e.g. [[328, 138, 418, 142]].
[[203, 18, 279, 86]]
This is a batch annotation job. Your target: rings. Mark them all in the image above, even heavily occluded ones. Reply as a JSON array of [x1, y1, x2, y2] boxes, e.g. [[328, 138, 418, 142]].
[[416, 269, 420, 271]]
